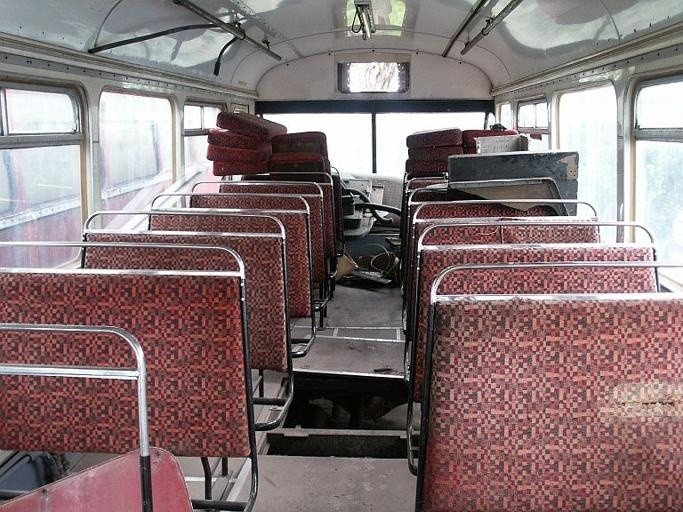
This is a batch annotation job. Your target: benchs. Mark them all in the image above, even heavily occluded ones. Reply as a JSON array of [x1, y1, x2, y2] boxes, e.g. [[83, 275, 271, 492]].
[[150, 175, 341, 359], [80, 210, 296, 475], [1, 324, 195, 512], [413, 265, 682, 511], [401, 174, 659, 475], [1, 242, 258, 511]]
